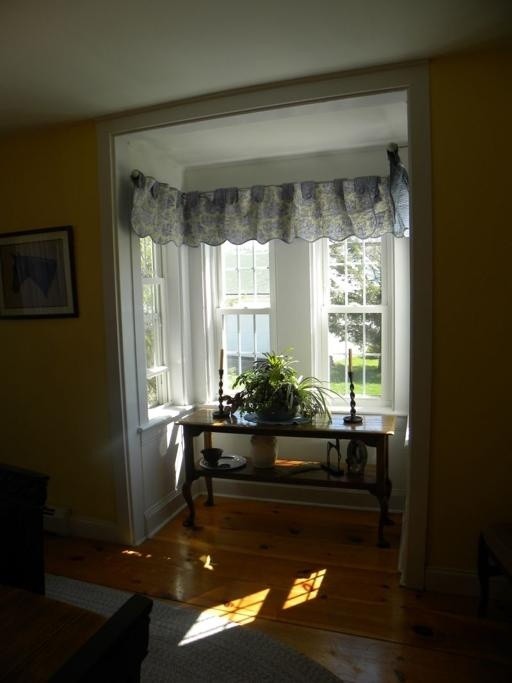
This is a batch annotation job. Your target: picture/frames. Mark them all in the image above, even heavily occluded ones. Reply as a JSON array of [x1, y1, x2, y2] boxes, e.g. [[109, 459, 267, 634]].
[[1, 225, 80, 321]]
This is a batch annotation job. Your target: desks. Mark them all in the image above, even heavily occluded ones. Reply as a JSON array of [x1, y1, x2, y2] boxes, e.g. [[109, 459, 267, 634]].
[[174, 407, 395, 547], [0, 585, 109, 683]]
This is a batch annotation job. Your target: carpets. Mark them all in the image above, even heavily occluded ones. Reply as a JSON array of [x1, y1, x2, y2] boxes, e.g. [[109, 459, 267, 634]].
[[43, 574, 346, 682]]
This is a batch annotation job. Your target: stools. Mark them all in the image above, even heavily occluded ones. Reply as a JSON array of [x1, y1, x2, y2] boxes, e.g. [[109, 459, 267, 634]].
[[477, 519, 511, 618]]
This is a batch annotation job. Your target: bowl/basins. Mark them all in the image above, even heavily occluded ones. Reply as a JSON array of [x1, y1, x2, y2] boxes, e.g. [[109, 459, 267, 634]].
[[201, 448, 223, 462]]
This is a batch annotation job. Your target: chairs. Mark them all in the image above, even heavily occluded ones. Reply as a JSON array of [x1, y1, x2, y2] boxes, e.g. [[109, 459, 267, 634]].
[[0, 463, 49, 597], [47, 592, 151, 682]]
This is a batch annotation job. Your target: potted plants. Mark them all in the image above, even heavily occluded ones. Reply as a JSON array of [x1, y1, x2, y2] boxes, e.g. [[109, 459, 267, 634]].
[[223, 351, 344, 425]]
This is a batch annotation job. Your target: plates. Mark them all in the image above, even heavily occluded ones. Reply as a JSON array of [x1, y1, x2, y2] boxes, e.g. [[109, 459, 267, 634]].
[[199, 452, 247, 471]]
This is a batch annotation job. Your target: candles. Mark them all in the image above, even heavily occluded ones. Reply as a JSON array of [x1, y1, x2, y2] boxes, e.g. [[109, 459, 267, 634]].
[[349, 347, 352, 374], [220, 349, 223, 370]]
